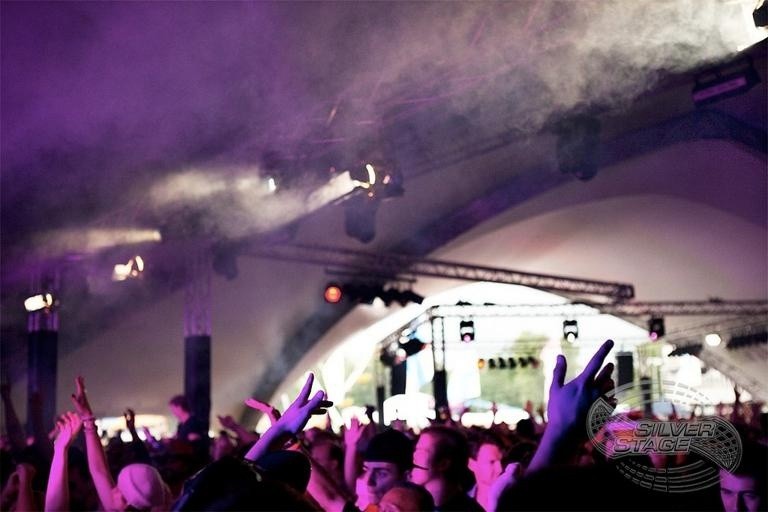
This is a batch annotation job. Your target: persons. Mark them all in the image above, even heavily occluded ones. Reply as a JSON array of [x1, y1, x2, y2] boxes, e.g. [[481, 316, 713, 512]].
[[1, 340, 766, 512]]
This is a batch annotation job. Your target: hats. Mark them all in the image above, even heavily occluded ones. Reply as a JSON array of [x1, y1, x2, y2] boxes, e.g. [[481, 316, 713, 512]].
[[252, 449, 310, 496], [360, 429, 412, 471], [118, 462, 164, 510]]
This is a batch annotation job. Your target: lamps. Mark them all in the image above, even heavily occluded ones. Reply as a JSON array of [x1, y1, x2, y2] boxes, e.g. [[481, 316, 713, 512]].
[[562, 319, 580, 342], [459, 317, 476, 343], [471, 352, 543, 372], [647, 313, 666, 340], [324, 271, 425, 310]]
